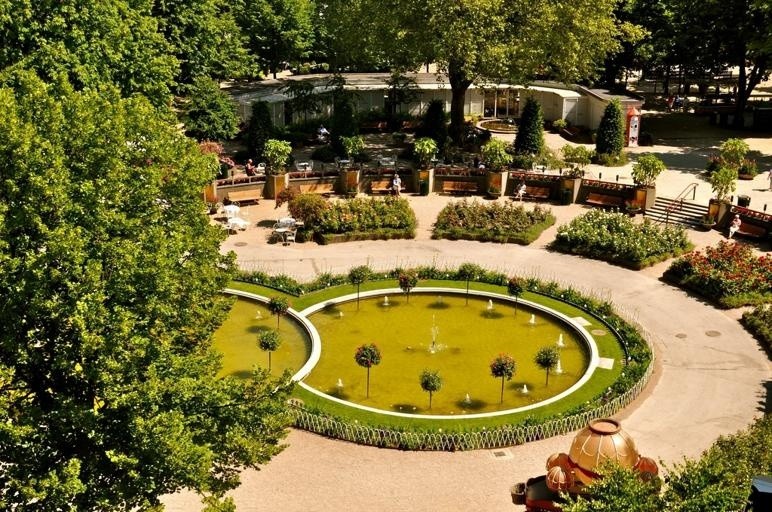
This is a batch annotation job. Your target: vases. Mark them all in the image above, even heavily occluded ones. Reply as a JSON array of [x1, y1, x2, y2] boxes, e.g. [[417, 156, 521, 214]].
[[346, 191, 358, 198], [487, 191, 502, 199], [625, 206, 643, 216]]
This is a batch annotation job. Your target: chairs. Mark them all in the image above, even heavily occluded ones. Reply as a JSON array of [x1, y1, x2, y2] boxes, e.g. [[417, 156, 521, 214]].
[[218, 201, 301, 246]]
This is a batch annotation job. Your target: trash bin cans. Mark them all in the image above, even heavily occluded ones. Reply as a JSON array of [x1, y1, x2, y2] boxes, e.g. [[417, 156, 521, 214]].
[[420, 180, 429, 196], [738, 195, 751, 207], [561, 188, 571, 205]]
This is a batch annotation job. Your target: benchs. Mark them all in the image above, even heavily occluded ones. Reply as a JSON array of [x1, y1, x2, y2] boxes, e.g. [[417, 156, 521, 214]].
[[299, 184, 335, 198], [226, 188, 264, 206], [442, 181, 478, 196], [370, 180, 407, 194], [730, 220, 765, 239], [585, 193, 623, 212], [514, 186, 551, 202]]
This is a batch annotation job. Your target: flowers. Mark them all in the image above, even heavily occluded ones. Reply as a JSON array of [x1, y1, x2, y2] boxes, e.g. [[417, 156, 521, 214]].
[[624, 200, 641, 209], [343, 176, 359, 193], [487, 180, 502, 193]]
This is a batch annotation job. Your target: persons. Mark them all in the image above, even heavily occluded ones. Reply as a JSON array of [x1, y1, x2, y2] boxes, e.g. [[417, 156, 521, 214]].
[[473, 154, 481, 169], [515, 179, 527, 202], [393, 173, 402, 197], [667, 94, 689, 113], [767, 169, 772, 189], [376, 151, 383, 166], [727, 214, 742, 239], [245, 158, 256, 176], [321, 125, 330, 143]]
